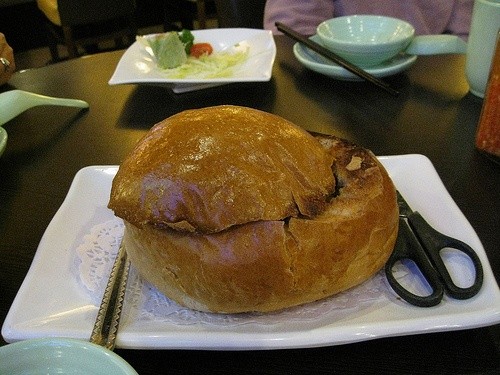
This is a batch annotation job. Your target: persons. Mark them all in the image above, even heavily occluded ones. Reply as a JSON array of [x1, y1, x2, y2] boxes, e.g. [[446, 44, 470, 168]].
[[263, 1, 473, 38], [0, 33, 15, 87]]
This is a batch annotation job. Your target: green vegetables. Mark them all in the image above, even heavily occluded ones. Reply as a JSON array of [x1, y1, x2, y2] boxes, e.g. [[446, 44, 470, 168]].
[[175, 29, 194, 56]]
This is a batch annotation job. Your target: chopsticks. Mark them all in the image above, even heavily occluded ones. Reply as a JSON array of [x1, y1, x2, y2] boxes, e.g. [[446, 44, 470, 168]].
[[274, 21, 404, 101]]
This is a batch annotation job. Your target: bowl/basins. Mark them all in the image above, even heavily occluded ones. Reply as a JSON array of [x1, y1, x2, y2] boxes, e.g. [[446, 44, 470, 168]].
[[0, 337, 138, 375], [0, 126, 8, 157], [317, 14, 415, 67]]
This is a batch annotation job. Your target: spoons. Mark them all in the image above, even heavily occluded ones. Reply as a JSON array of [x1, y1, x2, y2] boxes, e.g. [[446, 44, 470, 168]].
[[405, 34, 466, 55], [0, 89, 89, 127]]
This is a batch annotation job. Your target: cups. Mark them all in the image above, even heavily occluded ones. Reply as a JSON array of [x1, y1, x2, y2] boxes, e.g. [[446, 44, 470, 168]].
[[464, 0, 500, 97]]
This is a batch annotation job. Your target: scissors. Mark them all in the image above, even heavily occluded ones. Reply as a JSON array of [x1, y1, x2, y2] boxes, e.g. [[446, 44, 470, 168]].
[[385, 188, 485, 308]]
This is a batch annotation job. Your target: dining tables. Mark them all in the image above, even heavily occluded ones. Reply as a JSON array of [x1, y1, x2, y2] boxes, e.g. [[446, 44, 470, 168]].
[[0, 47, 499, 375]]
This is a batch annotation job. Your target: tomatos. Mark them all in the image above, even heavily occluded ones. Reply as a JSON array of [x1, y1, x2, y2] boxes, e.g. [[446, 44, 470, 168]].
[[192, 43, 213, 57]]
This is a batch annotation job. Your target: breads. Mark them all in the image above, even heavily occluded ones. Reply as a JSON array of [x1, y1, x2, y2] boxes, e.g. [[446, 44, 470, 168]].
[[108, 104, 399, 313]]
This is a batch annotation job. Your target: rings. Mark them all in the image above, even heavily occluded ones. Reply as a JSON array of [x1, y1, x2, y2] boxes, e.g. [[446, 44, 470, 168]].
[[0, 57, 10, 70]]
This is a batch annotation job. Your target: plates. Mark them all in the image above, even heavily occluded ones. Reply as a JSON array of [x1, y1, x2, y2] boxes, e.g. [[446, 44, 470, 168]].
[[108, 28, 277, 86], [293, 35, 417, 82], [1, 153, 500, 354]]
